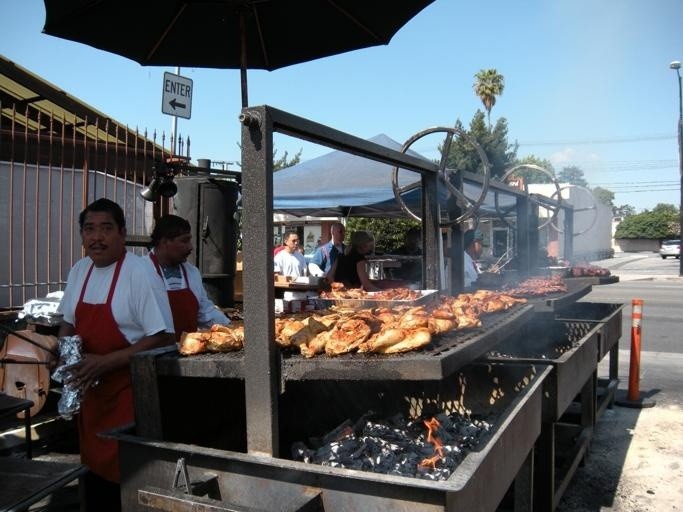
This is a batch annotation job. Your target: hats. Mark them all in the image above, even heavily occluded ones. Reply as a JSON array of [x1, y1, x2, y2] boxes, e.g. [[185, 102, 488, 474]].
[[465, 229, 483, 246]]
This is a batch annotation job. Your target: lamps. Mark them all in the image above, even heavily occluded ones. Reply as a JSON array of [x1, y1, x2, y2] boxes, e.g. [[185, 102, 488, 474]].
[[139, 158, 178, 203]]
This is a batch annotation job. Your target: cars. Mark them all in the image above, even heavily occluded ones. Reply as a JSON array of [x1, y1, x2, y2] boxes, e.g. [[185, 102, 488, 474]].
[[659, 239, 680, 259]]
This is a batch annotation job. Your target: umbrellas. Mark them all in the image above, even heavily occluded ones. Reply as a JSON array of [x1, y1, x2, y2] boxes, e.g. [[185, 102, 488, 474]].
[[40, 0, 434, 108]]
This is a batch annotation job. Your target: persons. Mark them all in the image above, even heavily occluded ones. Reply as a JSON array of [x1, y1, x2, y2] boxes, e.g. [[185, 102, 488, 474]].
[[50, 197, 175, 482], [272, 221, 380, 295], [387, 227, 423, 281], [445, 229, 483, 287], [143, 215, 230, 340]]
